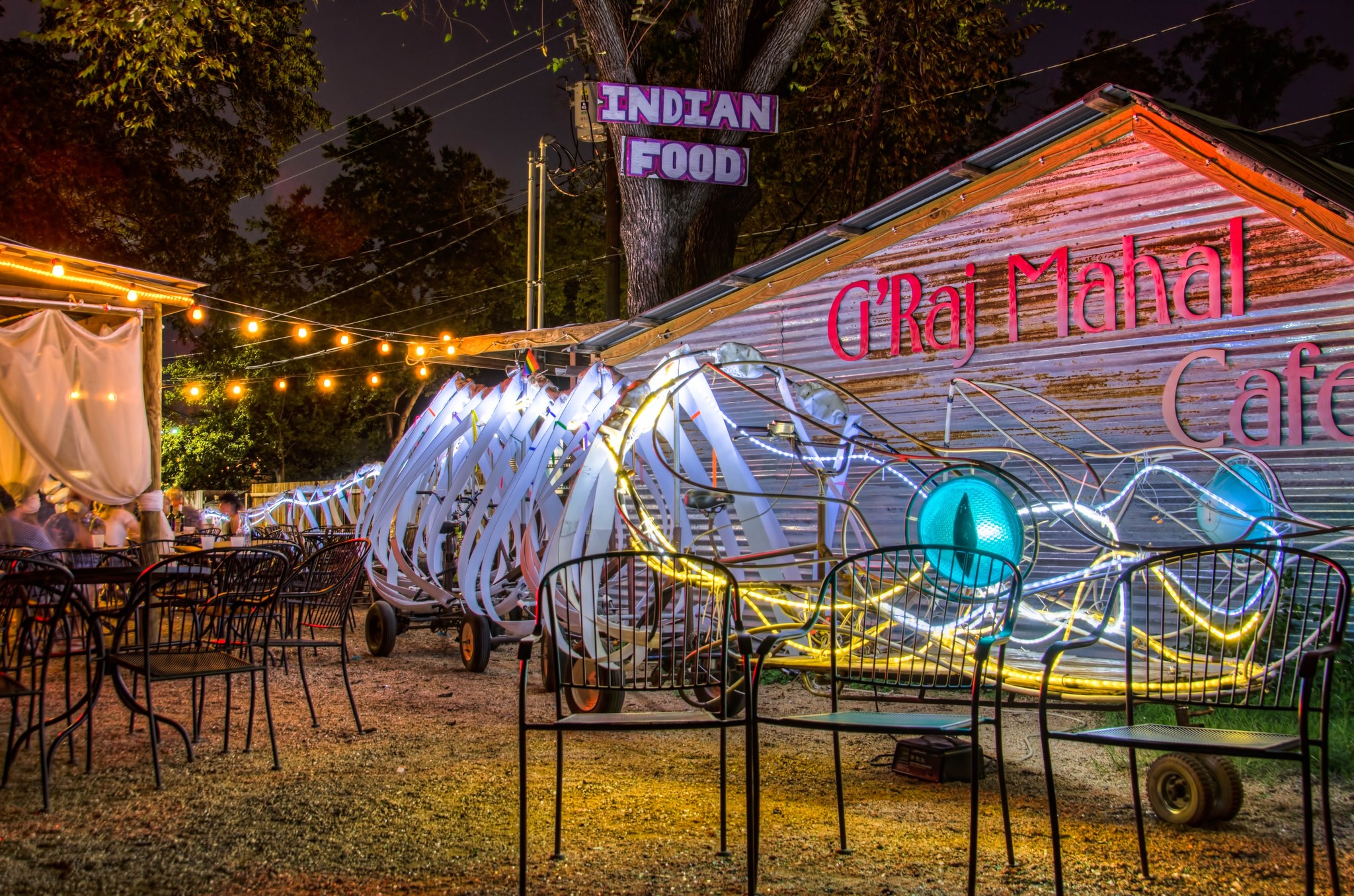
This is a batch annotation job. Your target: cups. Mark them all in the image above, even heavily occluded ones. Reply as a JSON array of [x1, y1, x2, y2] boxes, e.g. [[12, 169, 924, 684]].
[[230, 537, 245, 554], [201, 536, 215, 550], [92, 534, 105, 548]]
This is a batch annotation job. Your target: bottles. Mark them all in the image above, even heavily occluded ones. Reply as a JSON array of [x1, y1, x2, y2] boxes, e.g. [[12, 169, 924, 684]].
[[175, 504, 185, 533], [236, 510, 252, 548], [167, 502, 175, 532]]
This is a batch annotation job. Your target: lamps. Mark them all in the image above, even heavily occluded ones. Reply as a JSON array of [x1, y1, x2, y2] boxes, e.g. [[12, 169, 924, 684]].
[[505, 344, 523, 378]]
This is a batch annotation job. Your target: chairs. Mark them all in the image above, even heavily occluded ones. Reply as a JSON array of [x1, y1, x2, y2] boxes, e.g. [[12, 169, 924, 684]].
[[518, 551, 753, 896], [0, 524, 419, 813], [1039, 541, 1352, 896], [752, 544, 1022, 896]]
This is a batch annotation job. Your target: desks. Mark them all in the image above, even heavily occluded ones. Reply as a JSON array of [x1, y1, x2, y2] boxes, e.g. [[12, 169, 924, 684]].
[[1, 567, 214, 789], [175, 539, 296, 634], [90, 546, 140, 565]]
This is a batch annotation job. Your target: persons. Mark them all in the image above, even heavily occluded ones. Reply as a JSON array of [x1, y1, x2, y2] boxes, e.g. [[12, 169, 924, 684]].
[[95, 506, 141, 601], [0, 484, 76, 657], [42, 490, 106, 644], [218, 492, 257, 540], [24, 490, 56, 527], [167, 486, 202, 531], [162, 492, 183, 532]]
